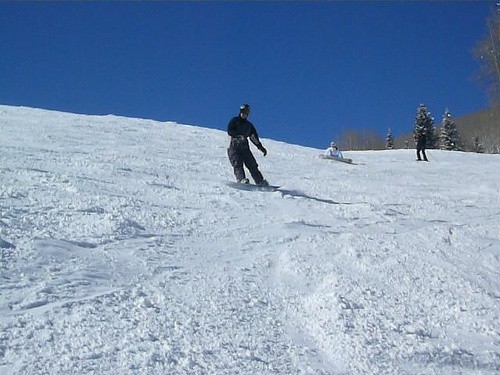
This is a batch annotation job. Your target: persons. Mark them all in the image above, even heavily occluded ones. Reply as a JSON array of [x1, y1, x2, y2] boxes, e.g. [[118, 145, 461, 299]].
[[417, 132, 428, 160], [325, 142, 343, 160], [227, 104, 270, 186]]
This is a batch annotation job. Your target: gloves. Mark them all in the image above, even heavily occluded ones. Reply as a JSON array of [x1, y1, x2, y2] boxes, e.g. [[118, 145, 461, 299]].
[[260, 146, 267, 156], [235, 134, 244, 143]]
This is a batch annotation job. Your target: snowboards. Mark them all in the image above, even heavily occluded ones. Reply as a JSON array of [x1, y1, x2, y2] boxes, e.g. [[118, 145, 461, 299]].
[[320, 154, 352, 163], [224, 180, 279, 192]]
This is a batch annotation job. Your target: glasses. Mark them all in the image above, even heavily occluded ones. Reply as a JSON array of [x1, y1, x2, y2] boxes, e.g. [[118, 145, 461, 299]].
[[240, 109, 249, 115]]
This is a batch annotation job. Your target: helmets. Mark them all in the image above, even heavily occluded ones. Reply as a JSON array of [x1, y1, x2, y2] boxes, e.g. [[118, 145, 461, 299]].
[[239, 104, 251, 117], [331, 142, 336, 146]]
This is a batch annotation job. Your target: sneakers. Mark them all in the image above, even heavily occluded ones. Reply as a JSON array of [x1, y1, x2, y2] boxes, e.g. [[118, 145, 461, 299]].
[[256, 179, 268, 187], [237, 178, 250, 184]]
